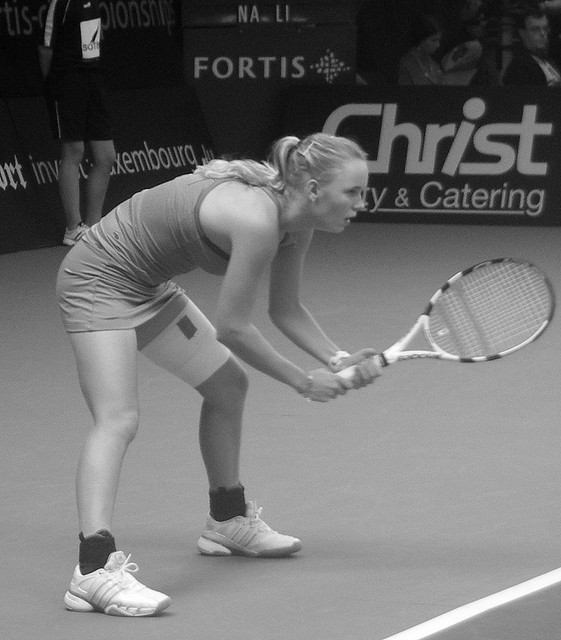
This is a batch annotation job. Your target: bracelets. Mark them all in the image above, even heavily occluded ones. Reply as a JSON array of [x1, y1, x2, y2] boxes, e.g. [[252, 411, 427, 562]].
[[301, 372, 312, 399], [329, 351, 351, 372]]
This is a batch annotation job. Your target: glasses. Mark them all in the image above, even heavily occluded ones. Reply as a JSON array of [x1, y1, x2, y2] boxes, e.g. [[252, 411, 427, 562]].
[[523, 25, 549, 32]]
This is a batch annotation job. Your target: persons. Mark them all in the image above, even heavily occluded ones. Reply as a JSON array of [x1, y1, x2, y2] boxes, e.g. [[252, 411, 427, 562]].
[[39, 0, 116, 246], [367, 49, 404, 86], [398, 16, 444, 88], [55, 130, 385, 618], [511, 9, 560, 87]]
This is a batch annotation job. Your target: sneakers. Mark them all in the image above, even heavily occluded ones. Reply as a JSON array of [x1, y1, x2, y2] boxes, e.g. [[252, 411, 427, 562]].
[[64, 551, 171, 617], [196, 500, 303, 558], [63, 221, 88, 245]]
